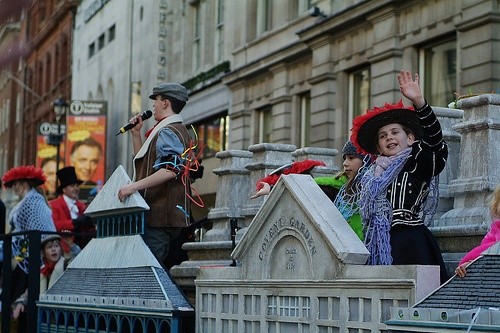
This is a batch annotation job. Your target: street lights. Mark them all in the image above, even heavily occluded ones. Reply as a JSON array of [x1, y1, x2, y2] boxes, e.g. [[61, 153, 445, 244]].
[[50, 94, 70, 199]]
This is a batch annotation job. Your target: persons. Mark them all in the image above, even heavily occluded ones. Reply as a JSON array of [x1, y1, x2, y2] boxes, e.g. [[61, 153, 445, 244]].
[[0, 165, 87, 333], [455, 180, 500, 278], [118, 83, 195, 271], [69, 138, 103, 186], [313, 141, 368, 241], [250, 69, 449, 286], [39, 157, 66, 196]]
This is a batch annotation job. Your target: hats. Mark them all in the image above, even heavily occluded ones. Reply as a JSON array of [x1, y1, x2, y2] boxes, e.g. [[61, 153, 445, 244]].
[[149, 84, 189, 103], [3, 166, 46, 187], [342, 141, 369, 163], [40, 234, 62, 250], [349, 102, 424, 157], [56, 166, 85, 193]]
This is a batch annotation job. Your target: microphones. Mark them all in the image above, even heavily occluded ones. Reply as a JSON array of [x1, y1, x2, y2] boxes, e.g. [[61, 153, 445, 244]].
[[116, 111, 152, 136]]
